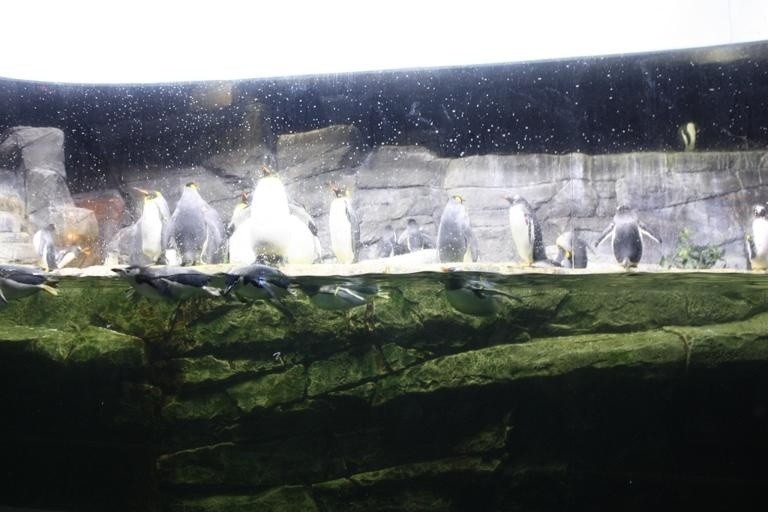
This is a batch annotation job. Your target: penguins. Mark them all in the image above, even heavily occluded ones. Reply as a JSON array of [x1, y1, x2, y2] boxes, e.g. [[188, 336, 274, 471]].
[[104, 164, 324, 271], [674, 113, 699, 152], [743, 201, 767, 273], [30, 221, 59, 270], [435, 194, 479, 263], [596, 202, 663, 270], [377, 222, 400, 259], [55, 245, 82, 270], [553, 222, 596, 270], [328, 186, 359, 263], [501, 195, 563, 269], [399, 218, 436, 252]]
